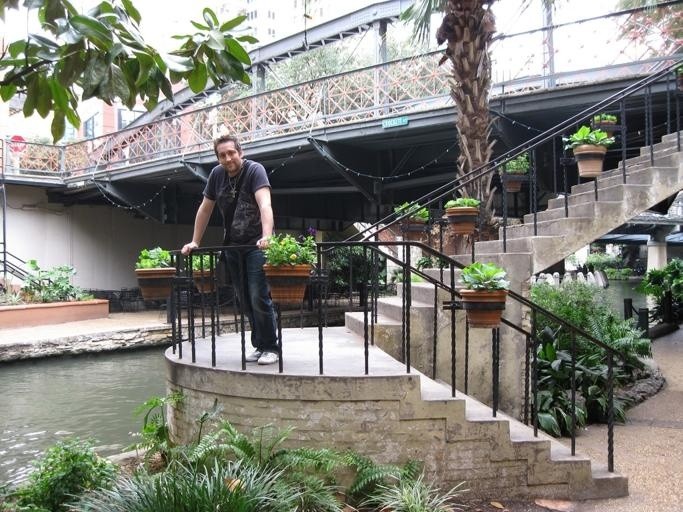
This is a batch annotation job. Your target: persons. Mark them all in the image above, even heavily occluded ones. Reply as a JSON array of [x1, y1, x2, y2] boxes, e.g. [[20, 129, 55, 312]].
[[179, 133, 279, 365]]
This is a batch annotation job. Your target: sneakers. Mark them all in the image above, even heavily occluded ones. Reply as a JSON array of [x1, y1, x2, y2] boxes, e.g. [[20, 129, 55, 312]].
[[246, 350, 262, 362], [258, 352, 280, 365]]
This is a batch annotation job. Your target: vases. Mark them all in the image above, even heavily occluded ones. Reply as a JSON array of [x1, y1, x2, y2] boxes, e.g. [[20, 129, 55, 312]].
[[262, 227, 317, 266], [262, 265, 312, 304]]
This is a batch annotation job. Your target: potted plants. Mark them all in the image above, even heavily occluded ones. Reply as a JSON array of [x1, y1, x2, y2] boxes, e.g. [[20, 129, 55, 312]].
[[590, 114, 617, 138], [444, 198, 481, 234], [135, 247, 177, 303], [184, 255, 217, 293], [562, 125, 615, 177], [458, 261, 511, 328], [394, 202, 429, 240], [498, 150, 531, 192]]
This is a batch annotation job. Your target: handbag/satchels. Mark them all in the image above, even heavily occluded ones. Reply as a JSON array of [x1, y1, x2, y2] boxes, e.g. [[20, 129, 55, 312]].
[[216, 256, 230, 283]]
